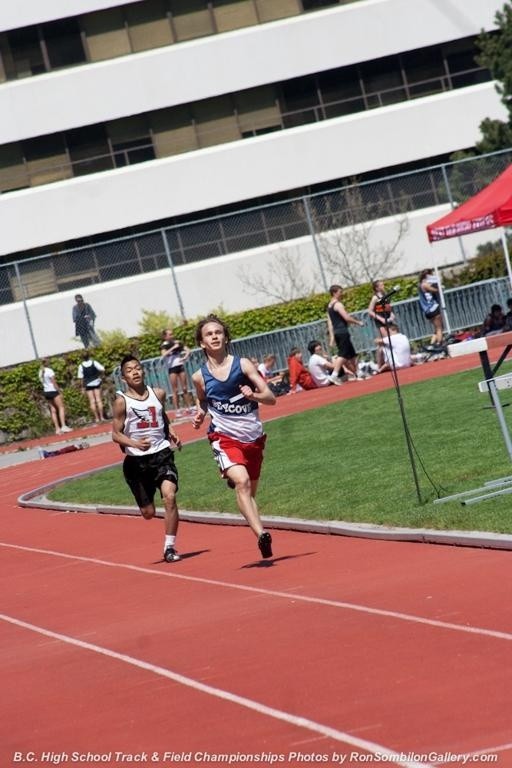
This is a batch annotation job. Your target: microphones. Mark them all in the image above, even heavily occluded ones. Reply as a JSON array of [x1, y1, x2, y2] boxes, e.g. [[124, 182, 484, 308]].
[[377, 285, 401, 306]]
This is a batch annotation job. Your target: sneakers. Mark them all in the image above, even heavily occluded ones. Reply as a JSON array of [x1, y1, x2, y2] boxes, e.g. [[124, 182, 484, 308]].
[[370, 364, 381, 375], [257, 533, 273, 559], [55, 431, 64, 436], [186, 408, 196, 414], [227, 478, 236, 489], [164, 546, 180, 563], [356, 371, 372, 380], [328, 374, 343, 385], [175, 409, 182, 417], [61, 426, 73, 432], [347, 374, 356, 381]]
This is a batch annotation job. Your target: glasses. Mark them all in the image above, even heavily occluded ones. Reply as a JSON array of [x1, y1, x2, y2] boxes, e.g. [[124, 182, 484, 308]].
[[77, 299, 83, 302]]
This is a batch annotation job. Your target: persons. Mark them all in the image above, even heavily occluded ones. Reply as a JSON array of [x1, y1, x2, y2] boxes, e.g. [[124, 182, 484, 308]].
[[191, 315, 278, 557], [287, 348, 344, 395], [419, 326, 479, 358], [39, 358, 73, 434], [74, 295, 101, 349], [419, 268, 446, 345], [480, 303, 506, 336], [78, 349, 106, 423], [506, 298, 512, 332], [259, 354, 292, 393], [367, 280, 399, 338], [309, 340, 364, 386], [160, 329, 190, 416], [373, 323, 411, 373], [251, 356, 283, 398], [327, 285, 373, 380], [112, 354, 185, 561]]
